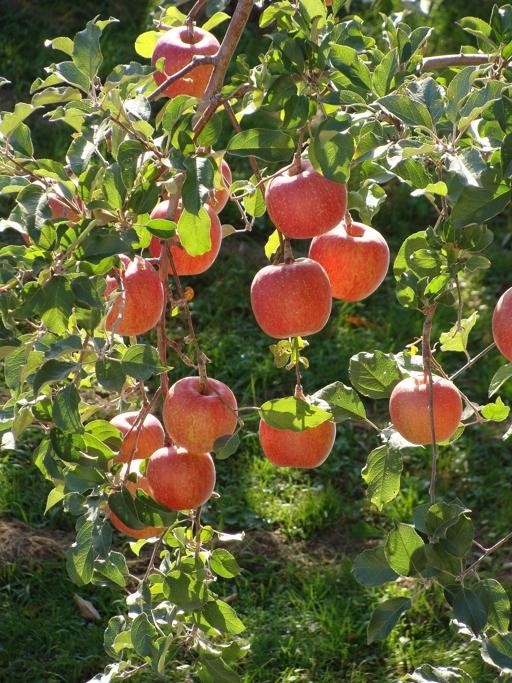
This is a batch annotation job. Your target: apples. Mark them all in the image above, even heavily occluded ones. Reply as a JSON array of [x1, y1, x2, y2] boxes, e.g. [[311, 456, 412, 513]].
[[22, 148, 235, 338], [107, 475, 166, 541], [389, 374, 463, 448], [150, 23, 221, 98], [162, 374, 240, 457], [144, 445, 219, 511], [309, 220, 391, 303], [248, 258, 334, 338], [107, 410, 164, 466], [263, 156, 348, 242], [257, 397, 338, 471], [490, 283, 512, 364]]
